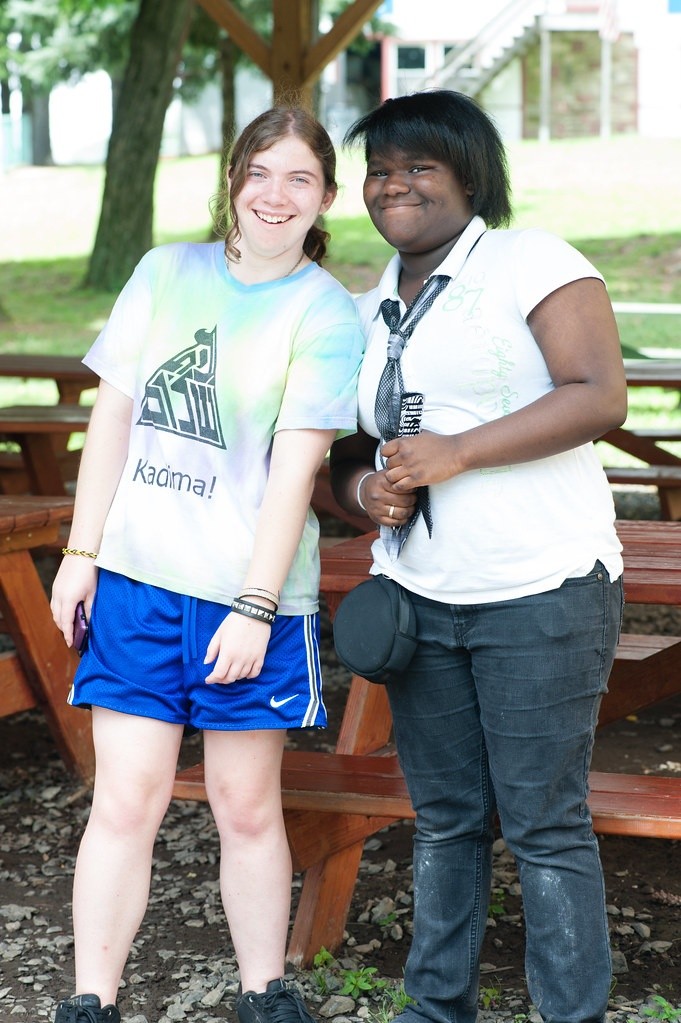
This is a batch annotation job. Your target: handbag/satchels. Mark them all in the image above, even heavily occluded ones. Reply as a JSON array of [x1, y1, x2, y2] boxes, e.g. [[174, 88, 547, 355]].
[[332, 575, 422, 687]]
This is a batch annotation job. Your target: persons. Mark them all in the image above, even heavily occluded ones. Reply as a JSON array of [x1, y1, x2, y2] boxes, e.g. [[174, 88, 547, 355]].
[[331, 88, 627, 1023], [47, 105, 364, 1023]]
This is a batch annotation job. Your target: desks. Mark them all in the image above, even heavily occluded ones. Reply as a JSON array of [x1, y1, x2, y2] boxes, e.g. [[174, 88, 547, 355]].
[[593, 357, 681, 522], [2, 497, 96, 796], [1, 352, 101, 498], [168, 517, 681, 969]]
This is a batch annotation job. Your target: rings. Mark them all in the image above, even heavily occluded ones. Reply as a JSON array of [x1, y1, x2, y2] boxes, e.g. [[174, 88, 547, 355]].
[[387, 505, 394, 519]]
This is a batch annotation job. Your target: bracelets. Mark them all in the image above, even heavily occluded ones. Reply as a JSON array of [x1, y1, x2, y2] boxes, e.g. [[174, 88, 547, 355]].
[[62, 547, 98, 559], [357, 472, 376, 513], [238, 587, 279, 610], [228, 596, 276, 627]]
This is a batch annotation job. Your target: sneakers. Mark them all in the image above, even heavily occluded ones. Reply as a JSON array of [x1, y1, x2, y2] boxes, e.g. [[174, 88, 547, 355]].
[[53, 979, 315, 1023]]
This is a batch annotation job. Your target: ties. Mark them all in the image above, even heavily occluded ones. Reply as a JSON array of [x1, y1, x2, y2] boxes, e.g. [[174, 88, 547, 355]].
[[379, 276, 433, 559]]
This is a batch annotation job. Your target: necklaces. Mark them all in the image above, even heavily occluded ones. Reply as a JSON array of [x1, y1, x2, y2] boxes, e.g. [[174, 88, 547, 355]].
[[283, 252, 307, 280]]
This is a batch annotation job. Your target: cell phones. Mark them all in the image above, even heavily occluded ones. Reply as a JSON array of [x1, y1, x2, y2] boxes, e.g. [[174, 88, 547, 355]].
[[75, 600, 88, 654]]
